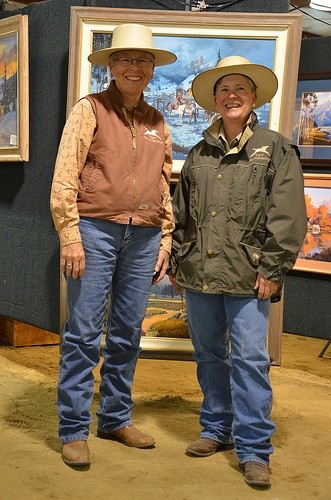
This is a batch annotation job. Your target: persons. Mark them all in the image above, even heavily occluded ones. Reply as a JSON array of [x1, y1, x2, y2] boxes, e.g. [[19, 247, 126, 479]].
[[167, 54, 308, 487], [49, 24, 176, 469]]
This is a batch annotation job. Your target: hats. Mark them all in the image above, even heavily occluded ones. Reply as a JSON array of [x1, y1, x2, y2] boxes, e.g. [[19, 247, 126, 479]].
[[87, 24, 177, 67], [191, 55, 278, 113]]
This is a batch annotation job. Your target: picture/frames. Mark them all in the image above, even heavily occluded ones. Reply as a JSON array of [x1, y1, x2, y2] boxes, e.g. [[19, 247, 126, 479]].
[[290, 172, 331, 276], [0, 14, 29, 162], [292, 71, 331, 167], [65, 6, 303, 184], [60, 198, 283, 365]]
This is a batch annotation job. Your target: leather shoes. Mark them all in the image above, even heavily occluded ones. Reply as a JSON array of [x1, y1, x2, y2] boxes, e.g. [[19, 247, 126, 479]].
[[186, 437, 235, 455], [62, 440, 91, 465], [97, 423, 156, 447], [239, 459, 272, 485]]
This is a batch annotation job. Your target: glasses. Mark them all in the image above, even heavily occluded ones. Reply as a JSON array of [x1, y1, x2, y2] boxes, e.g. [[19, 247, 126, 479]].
[[114, 58, 153, 66]]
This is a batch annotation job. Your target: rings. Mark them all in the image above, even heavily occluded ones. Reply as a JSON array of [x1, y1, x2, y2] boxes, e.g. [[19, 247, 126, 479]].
[[66, 264, 72, 266]]
